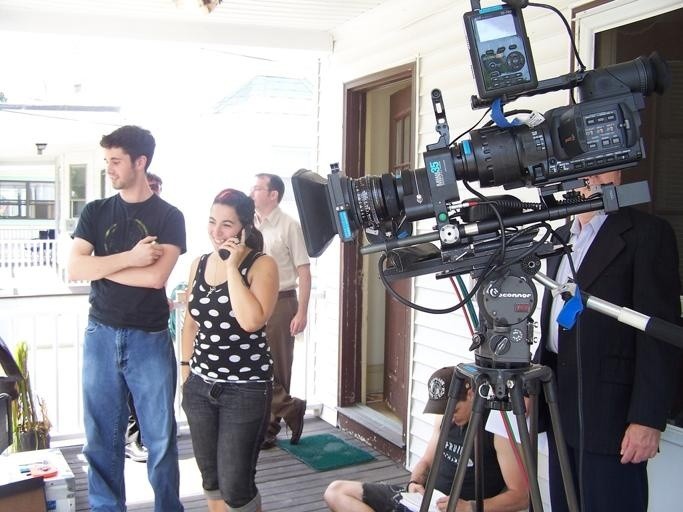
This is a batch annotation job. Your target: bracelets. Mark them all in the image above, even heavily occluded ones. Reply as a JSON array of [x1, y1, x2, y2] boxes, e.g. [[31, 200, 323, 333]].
[[178, 361, 191, 366], [405, 480, 419, 492]]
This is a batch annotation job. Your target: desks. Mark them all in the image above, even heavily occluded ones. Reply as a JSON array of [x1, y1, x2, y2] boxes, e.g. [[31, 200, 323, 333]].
[[0, 476, 47, 512], [8, 448, 76, 512]]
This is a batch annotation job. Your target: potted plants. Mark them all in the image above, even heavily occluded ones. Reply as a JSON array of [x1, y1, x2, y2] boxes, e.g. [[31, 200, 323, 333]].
[[12, 342, 51, 452]]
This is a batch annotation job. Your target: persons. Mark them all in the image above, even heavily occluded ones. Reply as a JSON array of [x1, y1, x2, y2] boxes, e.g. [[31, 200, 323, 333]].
[[182, 187, 279, 512], [67, 124, 186, 512], [521, 161, 676, 512], [322, 364, 532, 512], [125, 171, 179, 463], [248, 173, 306, 451]]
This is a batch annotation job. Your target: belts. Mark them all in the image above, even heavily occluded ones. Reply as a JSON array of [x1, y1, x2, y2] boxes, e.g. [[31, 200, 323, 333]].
[[276, 289, 296, 298]]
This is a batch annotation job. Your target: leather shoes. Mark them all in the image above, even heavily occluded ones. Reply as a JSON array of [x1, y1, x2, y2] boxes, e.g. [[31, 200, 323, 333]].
[[285, 400, 307, 445], [261, 431, 277, 450], [125, 442, 148, 462]]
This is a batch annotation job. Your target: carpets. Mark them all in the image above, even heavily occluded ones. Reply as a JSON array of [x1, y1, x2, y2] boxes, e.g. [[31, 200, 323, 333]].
[[275, 433, 376, 472]]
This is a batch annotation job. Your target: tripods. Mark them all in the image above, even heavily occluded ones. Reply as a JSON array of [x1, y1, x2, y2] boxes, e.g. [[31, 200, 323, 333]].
[[421, 360, 579, 511]]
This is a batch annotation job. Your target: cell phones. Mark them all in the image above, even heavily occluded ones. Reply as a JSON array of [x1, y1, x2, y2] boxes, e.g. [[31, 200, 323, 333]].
[[217, 223, 255, 262]]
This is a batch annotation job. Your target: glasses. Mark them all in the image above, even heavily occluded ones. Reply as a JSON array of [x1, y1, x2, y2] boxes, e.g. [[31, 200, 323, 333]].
[[150, 184, 162, 194], [249, 186, 269, 193]]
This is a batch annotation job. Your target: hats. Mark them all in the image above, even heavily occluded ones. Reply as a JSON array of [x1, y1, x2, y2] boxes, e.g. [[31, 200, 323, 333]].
[[423, 366, 471, 415]]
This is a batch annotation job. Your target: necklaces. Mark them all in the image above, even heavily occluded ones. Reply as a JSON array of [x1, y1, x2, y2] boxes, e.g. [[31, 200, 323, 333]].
[[202, 253, 229, 298]]
